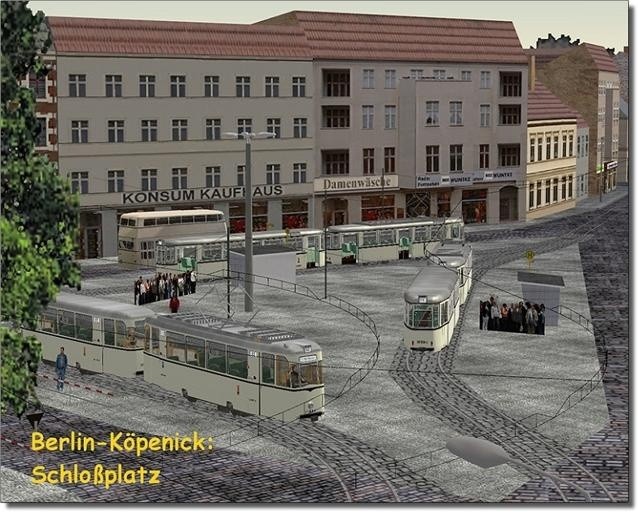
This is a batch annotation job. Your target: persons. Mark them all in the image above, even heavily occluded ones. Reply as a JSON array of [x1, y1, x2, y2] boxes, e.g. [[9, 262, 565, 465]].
[[479, 297, 547, 334], [134, 268, 198, 313], [53, 345, 67, 392]]
